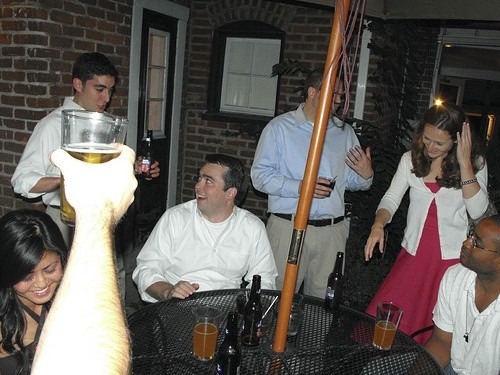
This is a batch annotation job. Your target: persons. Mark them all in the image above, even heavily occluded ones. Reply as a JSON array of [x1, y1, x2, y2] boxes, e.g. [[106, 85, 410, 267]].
[[250, 68, 374, 370], [351, 101, 490, 347], [132, 153, 280, 308], [363, 215, 500, 375], [0, 209, 68, 375], [29, 143, 138, 375], [10, 53, 160, 307]]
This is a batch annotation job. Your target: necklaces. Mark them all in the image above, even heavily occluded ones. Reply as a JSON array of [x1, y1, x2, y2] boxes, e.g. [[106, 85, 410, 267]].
[[463, 290, 480, 343]]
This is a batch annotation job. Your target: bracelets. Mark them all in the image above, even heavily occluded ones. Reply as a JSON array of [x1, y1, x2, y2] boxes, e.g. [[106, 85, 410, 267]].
[[460, 178, 477, 186]]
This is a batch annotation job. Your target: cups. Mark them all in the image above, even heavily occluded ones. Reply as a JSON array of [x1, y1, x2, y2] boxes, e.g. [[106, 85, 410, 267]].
[[318, 176, 336, 197], [372, 300, 405, 351], [287, 293, 304, 336], [60, 107, 129, 229], [237, 289, 251, 315], [191, 306, 222, 362], [260, 297, 274, 336]]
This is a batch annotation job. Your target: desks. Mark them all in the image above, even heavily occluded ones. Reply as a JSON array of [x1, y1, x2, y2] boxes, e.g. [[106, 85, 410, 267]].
[[125, 289, 447, 375]]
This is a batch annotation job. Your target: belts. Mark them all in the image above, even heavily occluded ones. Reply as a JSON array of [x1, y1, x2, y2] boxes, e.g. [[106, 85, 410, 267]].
[[273, 212, 344, 227]]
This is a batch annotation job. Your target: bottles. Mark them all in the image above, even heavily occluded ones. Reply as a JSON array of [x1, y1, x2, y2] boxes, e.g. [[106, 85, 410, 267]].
[[241, 275, 263, 347], [139, 129, 154, 181], [217, 310, 241, 375], [324, 251, 345, 310]]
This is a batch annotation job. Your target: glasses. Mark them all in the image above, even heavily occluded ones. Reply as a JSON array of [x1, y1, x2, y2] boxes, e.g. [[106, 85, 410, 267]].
[[467, 229, 497, 253]]
